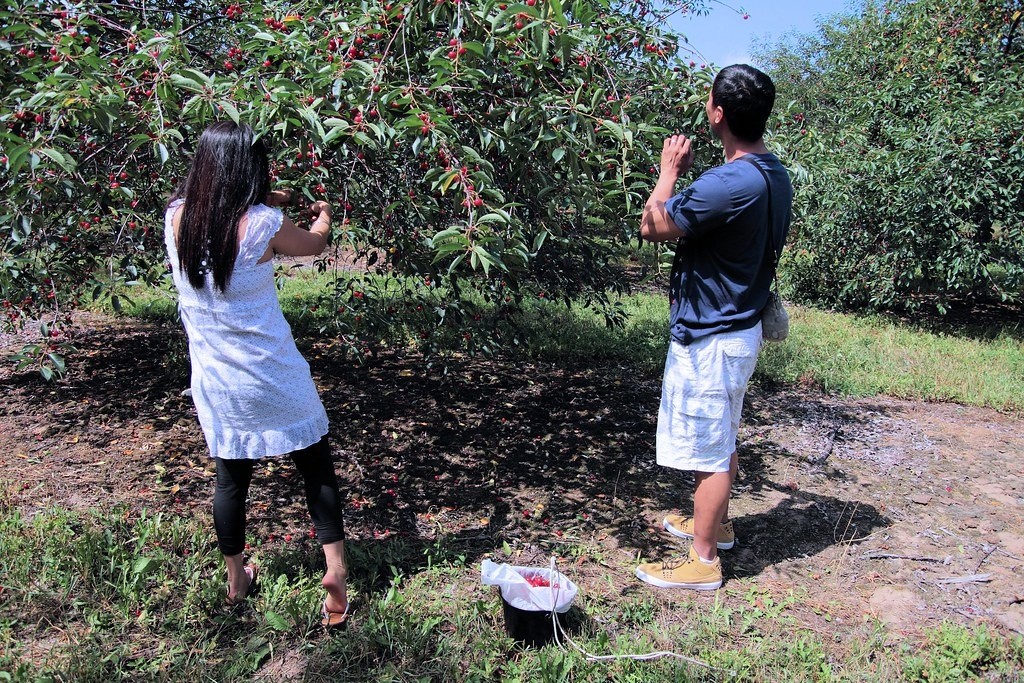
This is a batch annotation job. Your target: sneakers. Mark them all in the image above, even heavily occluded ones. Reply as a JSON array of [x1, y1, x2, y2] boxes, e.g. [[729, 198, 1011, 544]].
[[635, 544, 722, 590], [663, 514, 735, 549]]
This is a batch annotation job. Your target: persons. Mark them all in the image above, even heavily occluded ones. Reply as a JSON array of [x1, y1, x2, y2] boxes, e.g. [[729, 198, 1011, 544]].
[[635, 65, 792, 590], [164, 119, 347, 627]]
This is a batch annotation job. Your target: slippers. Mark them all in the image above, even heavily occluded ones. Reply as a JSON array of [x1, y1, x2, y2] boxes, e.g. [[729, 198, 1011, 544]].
[[321, 599, 350, 627], [226, 567, 254, 605]]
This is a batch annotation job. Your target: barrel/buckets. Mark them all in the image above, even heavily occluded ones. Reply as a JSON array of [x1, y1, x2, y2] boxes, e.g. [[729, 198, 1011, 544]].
[[498, 551, 577, 651]]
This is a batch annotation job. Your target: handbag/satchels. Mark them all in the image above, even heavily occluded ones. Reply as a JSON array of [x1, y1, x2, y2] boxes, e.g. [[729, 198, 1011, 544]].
[[760, 290, 789, 342]]
